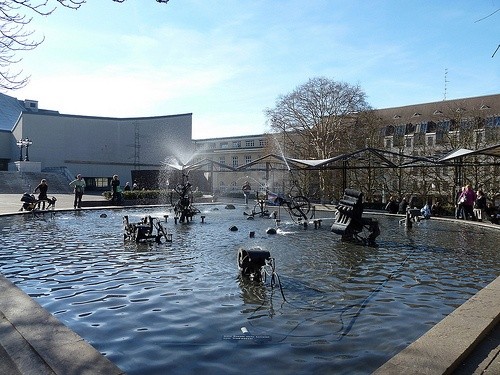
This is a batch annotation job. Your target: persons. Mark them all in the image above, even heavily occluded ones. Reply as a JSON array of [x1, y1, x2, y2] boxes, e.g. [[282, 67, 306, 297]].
[[132, 175, 155, 190], [124, 182, 131, 191], [420, 201, 442, 216], [455, 184, 486, 221], [242, 181, 251, 205], [487, 190, 500, 225], [111, 174, 120, 192], [34, 179, 47, 210], [385, 195, 408, 214], [71, 175, 86, 209]]
